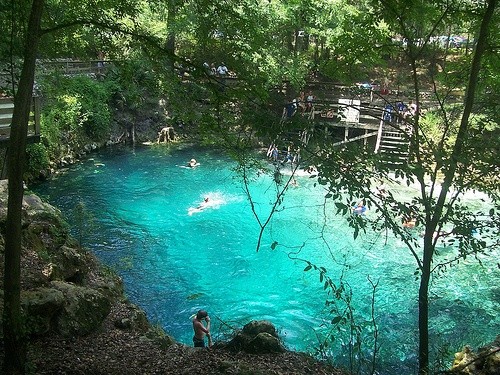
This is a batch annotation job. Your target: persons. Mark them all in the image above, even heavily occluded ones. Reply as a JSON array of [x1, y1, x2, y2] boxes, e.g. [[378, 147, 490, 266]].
[[403, 218, 417, 228], [96, 53, 107, 85], [192, 309, 212, 347], [385, 99, 417, 123], [291, 179, 298, 186], [270, 145, 295, 163], [187, 159, 197, 166], [297, 91, 317, 115], [203, 197, 210, 202], [202, 61, 229, 93], [352, 200, 367, 217]]
